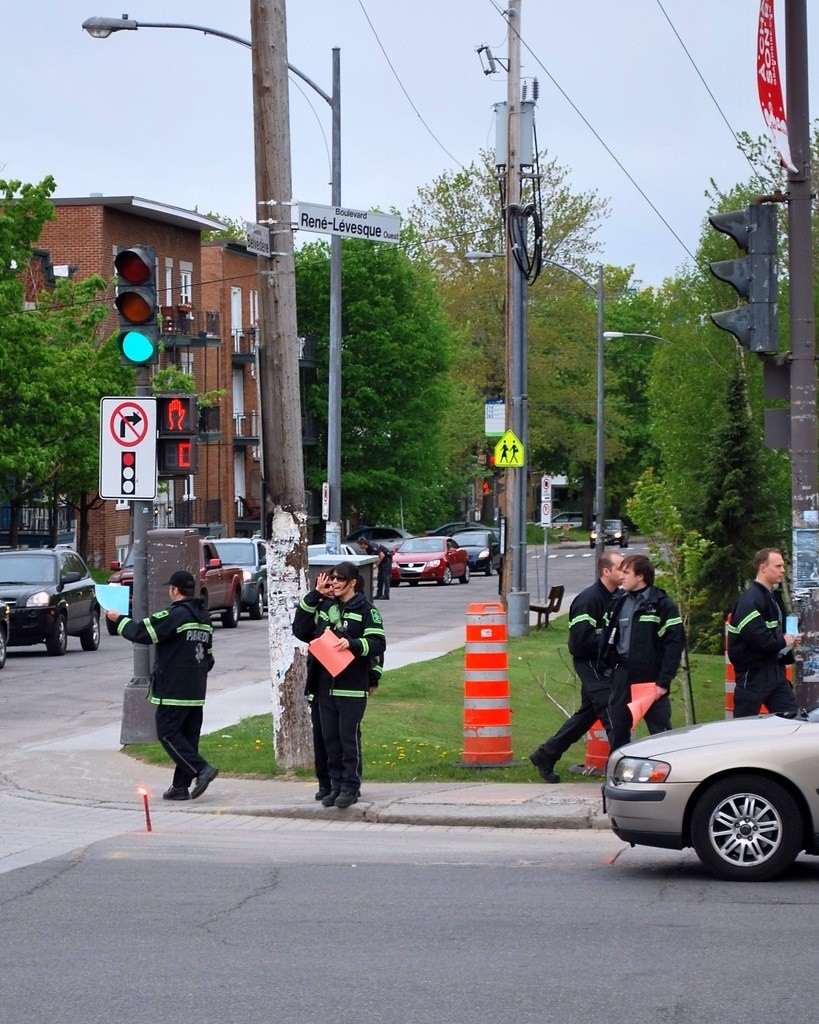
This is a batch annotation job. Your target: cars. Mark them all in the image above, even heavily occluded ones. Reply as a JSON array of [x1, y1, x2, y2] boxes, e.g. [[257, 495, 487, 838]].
[[601, 695, 819, 882], [588, 520, 629, 548], [307, 522, 501, 576], [390, 536, 470, 588], [106, 540, 244, 636], [0, 548, 101, 656]]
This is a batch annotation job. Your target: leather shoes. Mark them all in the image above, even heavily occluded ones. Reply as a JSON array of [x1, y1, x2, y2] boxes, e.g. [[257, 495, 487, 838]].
[[530, 750, 560, 783], [334, 791, 358, 807], [322, 790, 337, 806], [163, 784, 189, 800], [191, 765, 219, 798]]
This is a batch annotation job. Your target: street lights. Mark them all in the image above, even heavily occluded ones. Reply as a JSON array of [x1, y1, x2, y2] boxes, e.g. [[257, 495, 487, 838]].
[[82, 15, 341, 555], [464, 251, 604, 582]]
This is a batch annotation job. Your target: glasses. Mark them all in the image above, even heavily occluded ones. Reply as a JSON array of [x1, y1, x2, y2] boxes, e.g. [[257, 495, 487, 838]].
[[324, 584, 332, 588], [330, 574, 346, 582]]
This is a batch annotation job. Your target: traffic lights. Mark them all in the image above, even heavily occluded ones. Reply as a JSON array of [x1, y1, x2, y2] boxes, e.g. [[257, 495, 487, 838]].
[[115, 244, 159, 367], [471, 440, 506, 496], [156, 395, 199, 474], [709, 206, 779, 355]]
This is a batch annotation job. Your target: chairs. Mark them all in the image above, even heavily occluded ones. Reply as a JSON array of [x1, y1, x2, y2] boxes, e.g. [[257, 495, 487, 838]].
[[527, 585, 566, 627], [240, 495, 262, 520]]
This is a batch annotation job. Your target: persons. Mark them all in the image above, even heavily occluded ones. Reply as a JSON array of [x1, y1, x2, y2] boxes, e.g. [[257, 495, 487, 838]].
[[610, 551, 685, 756], [728, 548, 801, 715], [105, 570, 222, 802], [358, 536, 393, 602], [294, 559, 386, 809], [528, 549, 635, 785]]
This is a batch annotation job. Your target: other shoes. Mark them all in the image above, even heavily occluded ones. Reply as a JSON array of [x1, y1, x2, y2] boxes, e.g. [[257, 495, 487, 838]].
[[380, 595, 390, 599], [373, 595, 381, 599], [316, 788, 328, 799]]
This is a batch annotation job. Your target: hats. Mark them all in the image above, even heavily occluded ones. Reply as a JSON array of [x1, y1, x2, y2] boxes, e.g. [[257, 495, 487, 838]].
[[357, 537, 368, 543], [163, 571, 195, 589]]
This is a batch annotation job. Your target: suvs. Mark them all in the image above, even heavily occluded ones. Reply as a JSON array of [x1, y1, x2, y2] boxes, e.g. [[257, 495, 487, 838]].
[[536, 512, 596, 530], [203, 535, 268, 620]]
